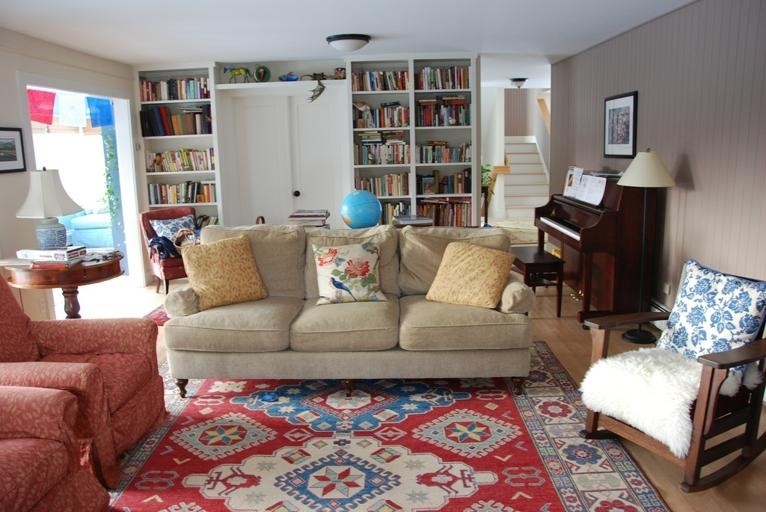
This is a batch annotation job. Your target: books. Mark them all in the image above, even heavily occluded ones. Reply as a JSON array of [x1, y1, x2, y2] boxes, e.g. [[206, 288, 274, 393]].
[[139, 77, 216, 206], [354, 66, 471, 227], [288, 209, 330, 228]]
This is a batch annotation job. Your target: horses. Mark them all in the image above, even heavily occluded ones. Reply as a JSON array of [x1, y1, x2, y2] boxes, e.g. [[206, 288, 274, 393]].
[[223, 66, 253, 83]]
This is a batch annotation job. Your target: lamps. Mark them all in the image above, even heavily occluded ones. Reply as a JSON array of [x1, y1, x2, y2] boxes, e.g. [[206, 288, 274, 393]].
[[327, 34, 371, 52], [16, 166, 84, 248], [509, 77, 529, 89], [616, 151, 676, 345]]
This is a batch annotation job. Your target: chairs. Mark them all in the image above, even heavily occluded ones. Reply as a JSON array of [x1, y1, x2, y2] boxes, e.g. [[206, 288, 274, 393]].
[[140, 206, 202, 296], [71, 209, 111, 248], [1, 386, 111, 512], [0, 272, 170, 490], [580, 314, 766, 494]]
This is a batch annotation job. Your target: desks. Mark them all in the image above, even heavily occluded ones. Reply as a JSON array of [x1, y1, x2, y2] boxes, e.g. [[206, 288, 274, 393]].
[[0, 249, 126, 319]]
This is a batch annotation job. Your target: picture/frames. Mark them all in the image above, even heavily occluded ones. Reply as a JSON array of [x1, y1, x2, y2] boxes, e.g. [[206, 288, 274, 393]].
[[0, 126, 27, 173], [604, 90, 637, 159]]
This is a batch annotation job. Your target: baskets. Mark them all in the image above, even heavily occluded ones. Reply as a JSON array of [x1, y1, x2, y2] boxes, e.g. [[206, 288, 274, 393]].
[[172, 227, 196, 256]]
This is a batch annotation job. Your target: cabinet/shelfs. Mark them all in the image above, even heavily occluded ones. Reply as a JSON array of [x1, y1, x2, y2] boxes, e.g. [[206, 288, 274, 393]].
[[132, 62, 225, 228], [345, 55, 481, 227]]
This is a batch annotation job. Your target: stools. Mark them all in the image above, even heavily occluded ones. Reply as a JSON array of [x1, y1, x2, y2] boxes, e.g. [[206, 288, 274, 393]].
[[510, 246, 567, 318]]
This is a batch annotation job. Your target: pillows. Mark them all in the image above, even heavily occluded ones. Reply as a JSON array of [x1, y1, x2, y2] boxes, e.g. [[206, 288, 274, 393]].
[[658, 259, 765, 374], [304, 225, 401, 298], [311, 241, 388, 305], [201, 223, 304, 300], [181, 235, 270, 312], [398, 223, 508, 296], [148, 214, 196, 242], [425, 241, 516, 309]]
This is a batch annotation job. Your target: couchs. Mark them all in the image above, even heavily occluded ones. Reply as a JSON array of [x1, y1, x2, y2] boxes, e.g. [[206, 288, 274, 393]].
[[162, 225, 536, 399]]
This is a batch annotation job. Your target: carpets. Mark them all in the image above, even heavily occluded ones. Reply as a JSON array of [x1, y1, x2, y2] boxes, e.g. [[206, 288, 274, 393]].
[[104, 340, 675, 512]]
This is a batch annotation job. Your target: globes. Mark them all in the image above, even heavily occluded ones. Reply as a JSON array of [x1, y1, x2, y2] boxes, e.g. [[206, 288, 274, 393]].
[[340, 190, 382, 229]]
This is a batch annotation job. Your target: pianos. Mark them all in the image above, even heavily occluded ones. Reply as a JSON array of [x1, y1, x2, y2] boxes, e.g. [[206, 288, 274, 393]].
[[535, 170, 656, 323]]
